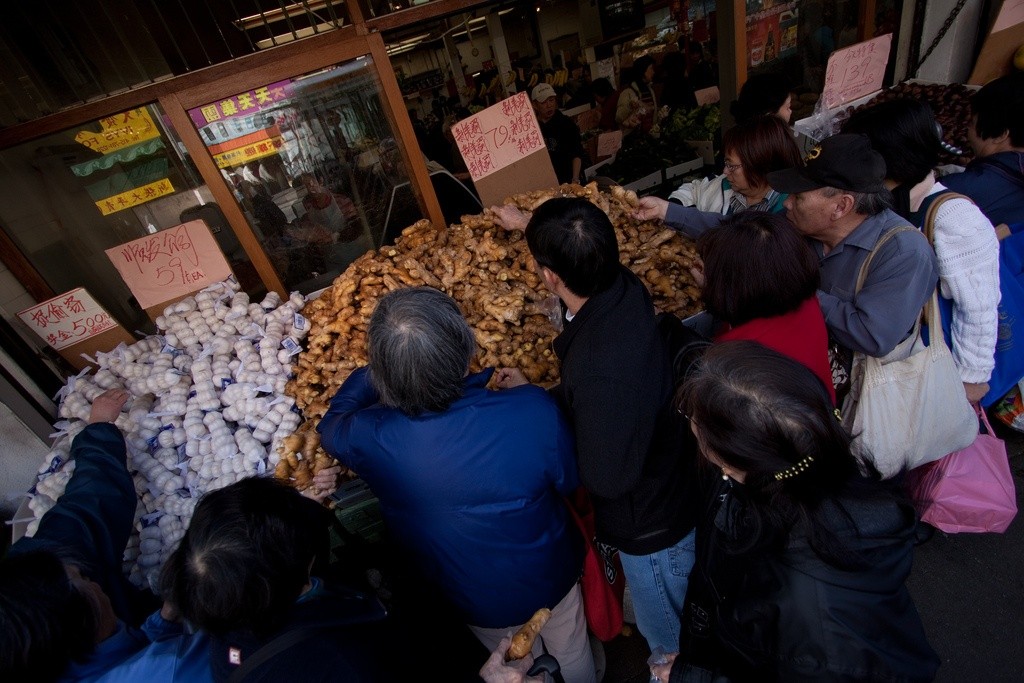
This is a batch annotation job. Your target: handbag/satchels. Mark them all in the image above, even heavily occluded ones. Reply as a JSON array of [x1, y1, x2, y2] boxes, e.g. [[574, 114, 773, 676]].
[[920, 192, 1024, 409], [838, 224, 979, 481], [564, 487, 625, 643], [911, 402, 1018, 535]]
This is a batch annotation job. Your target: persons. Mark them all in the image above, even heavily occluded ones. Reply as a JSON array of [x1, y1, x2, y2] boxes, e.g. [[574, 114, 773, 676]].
[[649, 306, 941, 683], [0, 537, 217, 683], [0, 388, 151, 627], [629, 133, 934, 546], [243, 11, 716, 302], [865, 97, 999, 403], [935, 73, 1024, 226], [490, 197, 708, 659], [667, 115, 805, 216], [315, 286, 606, 682], [160, 464, 545, 683], [728, 69, 793, 124], [698, 213, 837, 408]]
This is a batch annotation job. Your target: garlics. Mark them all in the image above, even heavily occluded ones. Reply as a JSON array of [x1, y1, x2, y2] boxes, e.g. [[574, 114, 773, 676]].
[[26, 282, 309, 593]]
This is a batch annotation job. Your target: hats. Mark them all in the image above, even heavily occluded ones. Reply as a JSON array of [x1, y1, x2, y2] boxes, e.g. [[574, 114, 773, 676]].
[[766, 130, 888, 194], [531, 82, 558, 103]]
